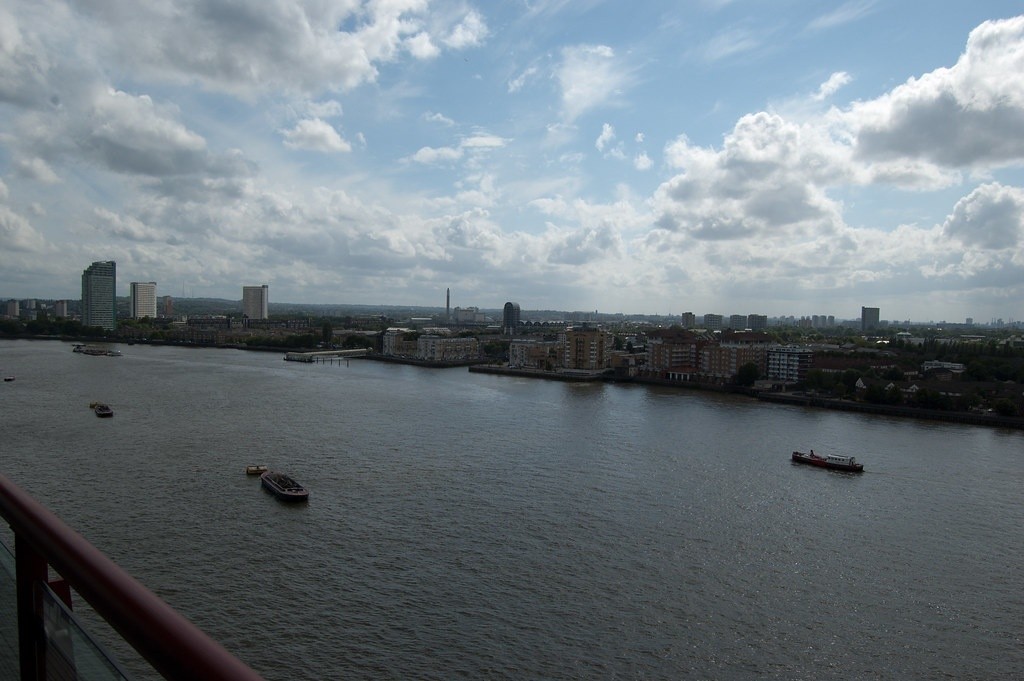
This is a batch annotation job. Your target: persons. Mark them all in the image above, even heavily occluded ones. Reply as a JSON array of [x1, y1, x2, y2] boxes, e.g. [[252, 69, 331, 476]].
[[810, 450, 814, 456]]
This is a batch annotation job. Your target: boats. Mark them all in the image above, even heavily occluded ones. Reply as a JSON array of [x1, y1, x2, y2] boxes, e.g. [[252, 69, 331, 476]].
[[790, 451, 863, 473], [260, 470, 309, 504], [71, 343, 121, 358], [94, 404, 113, 418]]
